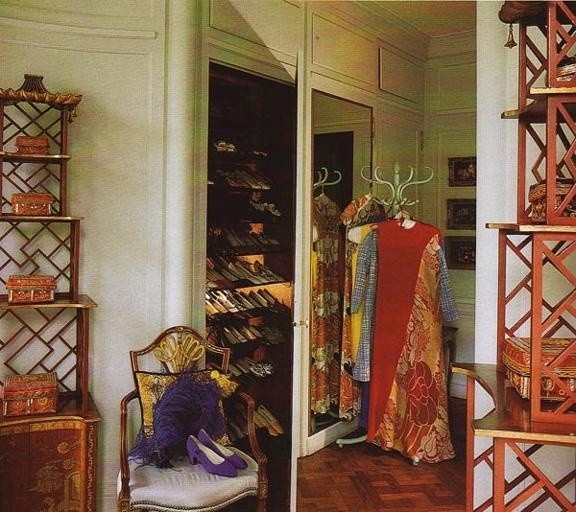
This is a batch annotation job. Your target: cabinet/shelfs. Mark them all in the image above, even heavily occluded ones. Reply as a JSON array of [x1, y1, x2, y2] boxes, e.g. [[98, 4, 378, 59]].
[[451, 0, 576, 512], [0, 73, 100, 511], [203, 56, 300, 461]]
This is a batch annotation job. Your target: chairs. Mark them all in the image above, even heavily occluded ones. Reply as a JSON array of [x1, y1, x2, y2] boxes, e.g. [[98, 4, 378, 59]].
[[115, 324, 270, 512]]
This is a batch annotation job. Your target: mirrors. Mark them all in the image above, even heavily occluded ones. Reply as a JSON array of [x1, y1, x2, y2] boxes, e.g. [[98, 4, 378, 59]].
[[305, 87, 378, 453]]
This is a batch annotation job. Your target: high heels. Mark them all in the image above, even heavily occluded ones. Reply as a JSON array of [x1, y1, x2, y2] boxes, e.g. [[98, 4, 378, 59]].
[[197, 428, 247, 469], [186, 435, 237, 477]]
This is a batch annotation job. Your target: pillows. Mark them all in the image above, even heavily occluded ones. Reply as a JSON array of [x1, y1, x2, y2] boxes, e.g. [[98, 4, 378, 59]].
[[133, 369, 232, 456]]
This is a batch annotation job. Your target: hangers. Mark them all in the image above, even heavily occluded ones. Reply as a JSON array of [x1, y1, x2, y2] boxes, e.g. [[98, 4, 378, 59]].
[[342, 180, 441, 239], [312, 185, 343, 213]]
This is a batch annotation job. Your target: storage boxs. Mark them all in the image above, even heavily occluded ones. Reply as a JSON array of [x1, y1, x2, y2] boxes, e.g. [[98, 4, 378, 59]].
[[2, 371, 60, 417], [12, 194, 55, 218], [15, 135, 50, 155], [500, 338, 575, 403], [6, 275, 57, 304], [527, 176, 575, 222]]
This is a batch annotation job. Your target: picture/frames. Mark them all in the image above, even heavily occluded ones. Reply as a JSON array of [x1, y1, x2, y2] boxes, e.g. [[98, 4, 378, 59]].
[[444, 234, 476, 270], [446, 197, 476, 230], [447, 155, 477, 187]]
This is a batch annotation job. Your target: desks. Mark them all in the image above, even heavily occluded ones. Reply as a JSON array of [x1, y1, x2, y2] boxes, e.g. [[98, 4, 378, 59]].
[[442, 324, 459, 417]]
